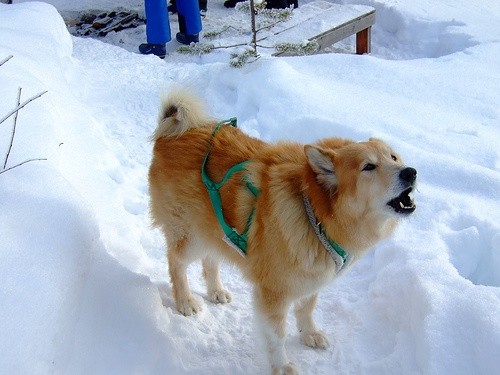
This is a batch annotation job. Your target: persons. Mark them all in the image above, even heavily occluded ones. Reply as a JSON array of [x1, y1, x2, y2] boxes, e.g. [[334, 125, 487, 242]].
[[138, 0, 202, 59]]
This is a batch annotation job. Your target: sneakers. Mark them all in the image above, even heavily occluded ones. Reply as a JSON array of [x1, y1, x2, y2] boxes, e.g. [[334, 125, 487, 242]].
[[139, 43, 166, 58], [176, 32, 199, 46]]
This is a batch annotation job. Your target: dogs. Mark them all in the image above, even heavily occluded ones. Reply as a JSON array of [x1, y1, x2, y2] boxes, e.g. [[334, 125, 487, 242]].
[[144, 84, 421, 375]]
